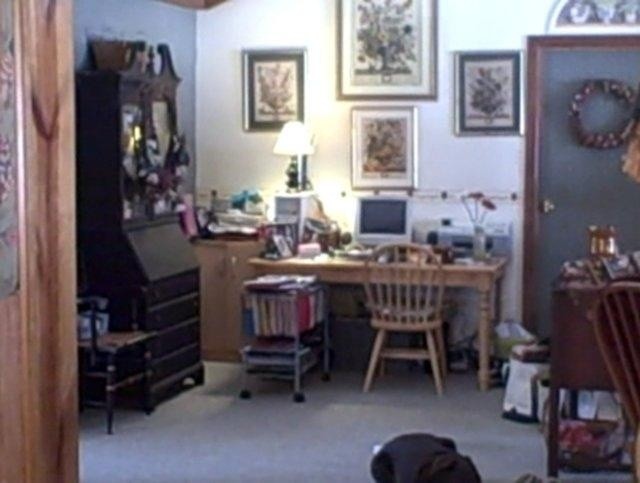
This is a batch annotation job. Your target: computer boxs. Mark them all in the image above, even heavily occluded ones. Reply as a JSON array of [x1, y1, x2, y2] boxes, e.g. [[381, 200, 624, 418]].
[[274, 191, 312, 244]]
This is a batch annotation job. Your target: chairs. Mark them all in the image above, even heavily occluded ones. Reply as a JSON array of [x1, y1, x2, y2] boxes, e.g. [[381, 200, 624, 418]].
[[77, 244, 160, 435], [362, 238, 451, 396], [591, 278, 640, 482]]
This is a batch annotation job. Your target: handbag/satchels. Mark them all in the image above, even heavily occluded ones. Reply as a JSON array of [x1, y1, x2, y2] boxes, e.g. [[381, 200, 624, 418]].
[[500, 356, 550, 423]]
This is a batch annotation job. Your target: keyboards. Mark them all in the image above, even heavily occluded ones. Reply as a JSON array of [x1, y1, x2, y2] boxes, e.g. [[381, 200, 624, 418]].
[[349, 249, 408, 262]]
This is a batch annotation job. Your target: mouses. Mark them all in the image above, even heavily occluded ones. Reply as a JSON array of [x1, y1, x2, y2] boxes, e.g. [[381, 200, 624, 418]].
[[378, 254, 392, 264]]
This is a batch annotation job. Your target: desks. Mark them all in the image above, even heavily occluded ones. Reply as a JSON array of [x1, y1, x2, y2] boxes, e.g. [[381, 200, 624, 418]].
[[240, 253, 510, 392]]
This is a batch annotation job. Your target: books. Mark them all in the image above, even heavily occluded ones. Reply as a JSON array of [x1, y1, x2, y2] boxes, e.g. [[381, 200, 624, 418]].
[[241, 272, 324, 377]]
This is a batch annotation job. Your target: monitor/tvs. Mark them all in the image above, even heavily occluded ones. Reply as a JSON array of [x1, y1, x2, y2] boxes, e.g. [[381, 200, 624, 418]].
[[353, 194, 411, 247]]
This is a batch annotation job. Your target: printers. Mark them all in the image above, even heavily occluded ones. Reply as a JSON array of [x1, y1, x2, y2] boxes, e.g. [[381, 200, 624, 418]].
[[414, 224, 512, 258]]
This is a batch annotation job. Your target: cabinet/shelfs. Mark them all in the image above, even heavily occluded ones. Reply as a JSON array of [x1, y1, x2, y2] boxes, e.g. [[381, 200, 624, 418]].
[[76, 69, 206, 413], [239, 280, 332, 402]]
